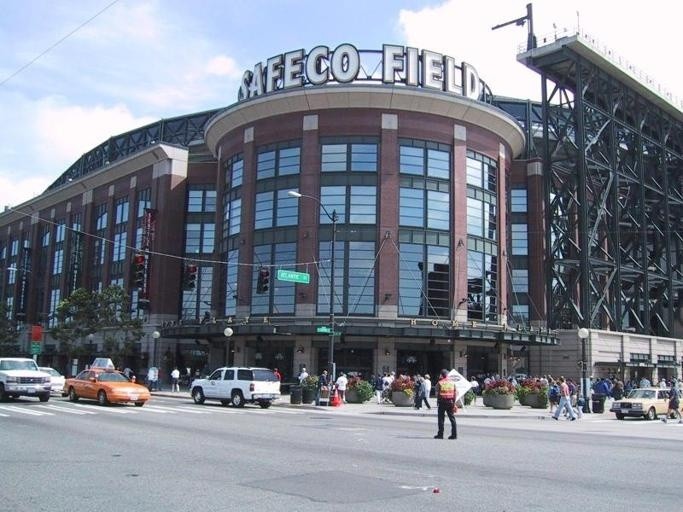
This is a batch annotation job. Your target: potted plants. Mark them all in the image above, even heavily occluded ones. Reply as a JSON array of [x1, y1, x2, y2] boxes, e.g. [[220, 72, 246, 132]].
[[302, 375, 319, 404], [457, 378, 548, 409], [388, 374, 416, 406], [344, 374, 373, 403]]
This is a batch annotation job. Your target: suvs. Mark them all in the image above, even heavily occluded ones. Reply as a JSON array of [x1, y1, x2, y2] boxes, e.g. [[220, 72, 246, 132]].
[[0, 358, 52, 403], [189, 365, 283, 409]]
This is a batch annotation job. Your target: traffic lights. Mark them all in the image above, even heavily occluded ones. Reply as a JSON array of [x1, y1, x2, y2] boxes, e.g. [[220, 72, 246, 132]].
[[185, 264, 197, 291], [255, 268, 270, 294], [131, 253, 146, 290]]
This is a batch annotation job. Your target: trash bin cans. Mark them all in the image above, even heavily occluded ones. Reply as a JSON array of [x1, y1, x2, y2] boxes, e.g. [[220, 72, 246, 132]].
[[290, 385, 301, 404], [592, 394, 604, 413]]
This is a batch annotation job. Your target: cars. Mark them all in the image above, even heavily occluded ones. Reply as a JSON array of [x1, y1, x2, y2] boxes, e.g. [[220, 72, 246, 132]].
[[611, 387, 682, 419], [63, 357, 152, 408], [32, 366, 67, 397]]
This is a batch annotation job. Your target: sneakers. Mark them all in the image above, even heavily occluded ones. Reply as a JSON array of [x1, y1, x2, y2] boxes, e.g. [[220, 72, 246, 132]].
[[448, 435, 457, 439], [434, 434, 443, 439]]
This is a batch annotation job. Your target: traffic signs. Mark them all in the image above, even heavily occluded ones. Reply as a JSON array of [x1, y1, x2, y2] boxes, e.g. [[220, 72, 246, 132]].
[[277, 270, 310, 284]]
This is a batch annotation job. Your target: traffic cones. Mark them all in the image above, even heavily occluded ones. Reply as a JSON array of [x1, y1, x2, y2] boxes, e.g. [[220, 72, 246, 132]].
[[331, 388, 342, 406]]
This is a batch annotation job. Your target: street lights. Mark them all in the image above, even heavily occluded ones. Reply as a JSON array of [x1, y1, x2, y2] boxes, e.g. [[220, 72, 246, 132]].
[[85, 334, 94, 367], [579, 327, 594, 412], [287, 190, 337, 386], [222, 327, 234, 367], [152, 331, 161, 366]]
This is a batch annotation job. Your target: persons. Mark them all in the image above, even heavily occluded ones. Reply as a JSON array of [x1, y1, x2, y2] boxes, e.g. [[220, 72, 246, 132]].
[[590, 376, 682, 424], [273, 369, 281, 381], [375, 371, 431, 409], [146, 364, 159, 389], [470, 373, 500, 404], [124, 366, 133, 380], [201, 363, 211, 379], [318, 370, 362, 400], [522, 375, 584, 421], [434, 370, 457, 439], [171, 366, 180, 393], [116, 366, 120, 371], [298, 368, 309, 383]]
